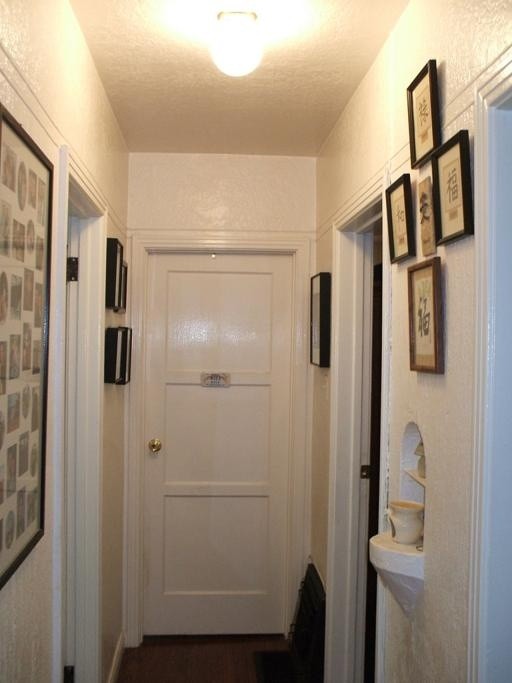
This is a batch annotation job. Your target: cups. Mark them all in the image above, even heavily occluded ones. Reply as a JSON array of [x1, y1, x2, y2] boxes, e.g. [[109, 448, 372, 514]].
[[388, 500, 425, 544]]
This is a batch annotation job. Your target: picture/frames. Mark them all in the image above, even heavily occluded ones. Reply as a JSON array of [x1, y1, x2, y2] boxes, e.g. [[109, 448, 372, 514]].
[[0, 102, 55, 593], [310, 272, 332, 368], [105, 238, 132, 386], [384, 60, 475, 375]]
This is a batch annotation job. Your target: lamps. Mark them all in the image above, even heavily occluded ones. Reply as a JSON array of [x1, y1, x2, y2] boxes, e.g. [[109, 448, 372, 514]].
[[208, 8, 266, 79]]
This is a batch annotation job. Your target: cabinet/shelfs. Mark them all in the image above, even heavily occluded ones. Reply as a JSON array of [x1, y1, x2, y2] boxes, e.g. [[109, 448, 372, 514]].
[[284, 563, 326, 683]]
